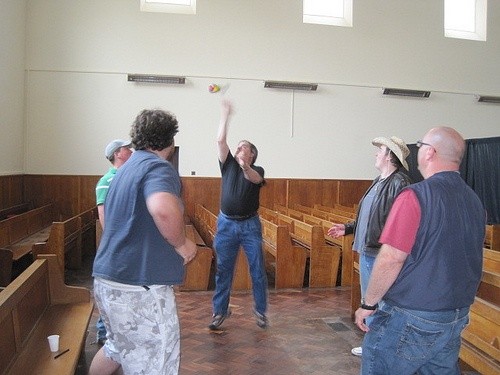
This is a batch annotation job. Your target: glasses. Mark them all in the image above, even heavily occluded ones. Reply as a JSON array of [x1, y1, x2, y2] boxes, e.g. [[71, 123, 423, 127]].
[[416, 140, 437, 153]]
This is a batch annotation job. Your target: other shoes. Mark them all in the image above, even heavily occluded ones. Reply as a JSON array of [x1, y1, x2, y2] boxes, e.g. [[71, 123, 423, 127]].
[[352, 346, 362, 356], [251, 309, 270, 328], [209, 310, 232, 330]]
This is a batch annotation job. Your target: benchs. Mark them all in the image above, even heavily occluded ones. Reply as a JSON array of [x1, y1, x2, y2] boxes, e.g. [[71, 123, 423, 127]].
[[0, 201, 500, 375]]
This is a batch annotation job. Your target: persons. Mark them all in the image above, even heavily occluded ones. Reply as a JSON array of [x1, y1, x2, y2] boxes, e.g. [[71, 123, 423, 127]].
[[328, 137, 413, 357], [354, 126, 487, 375], [209, 100, 268, 330], [94, 139, 132, 346], [88, 109, 198, 375]]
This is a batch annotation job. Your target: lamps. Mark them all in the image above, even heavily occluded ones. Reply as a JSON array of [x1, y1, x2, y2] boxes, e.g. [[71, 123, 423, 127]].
[[383, 88, 430, 98], [264, 81, 318, 91], [128, 74, 185, 84], [477, 95, 500, 103]]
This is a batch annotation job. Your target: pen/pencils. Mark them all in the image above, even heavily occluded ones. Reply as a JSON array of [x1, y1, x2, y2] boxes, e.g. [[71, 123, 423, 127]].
[[54, 348, 69, 359]]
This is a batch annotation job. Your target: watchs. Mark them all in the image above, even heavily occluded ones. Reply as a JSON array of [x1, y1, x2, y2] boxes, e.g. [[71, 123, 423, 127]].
[[360, 297, 379, 310]]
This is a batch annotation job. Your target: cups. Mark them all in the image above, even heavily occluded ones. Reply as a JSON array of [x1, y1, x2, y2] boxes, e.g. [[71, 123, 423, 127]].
[[47, 335, 59, 352]]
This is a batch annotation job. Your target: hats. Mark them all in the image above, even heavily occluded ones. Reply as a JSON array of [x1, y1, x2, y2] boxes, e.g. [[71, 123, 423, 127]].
[[372, 135, 410, 171], [105, 140, 131, 159]]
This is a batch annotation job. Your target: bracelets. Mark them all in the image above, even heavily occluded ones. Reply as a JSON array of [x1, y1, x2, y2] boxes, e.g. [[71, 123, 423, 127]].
[[244, 168, 249, 171]]
